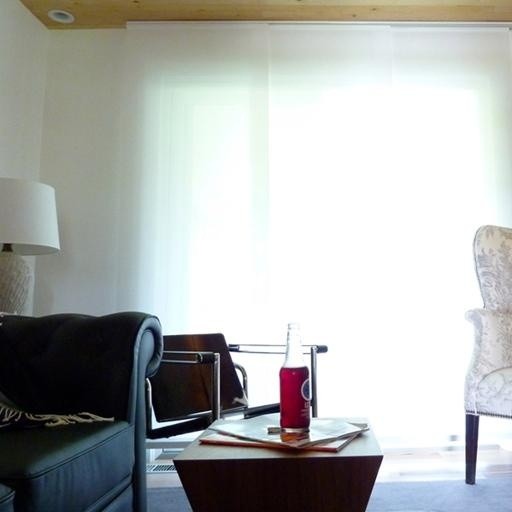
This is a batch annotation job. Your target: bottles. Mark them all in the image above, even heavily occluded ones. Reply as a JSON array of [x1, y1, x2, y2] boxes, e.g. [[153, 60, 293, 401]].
[[280, 325, 311, 432]]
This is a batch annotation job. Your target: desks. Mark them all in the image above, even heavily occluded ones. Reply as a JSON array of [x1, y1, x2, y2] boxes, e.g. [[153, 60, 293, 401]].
[[172, 416, 387, 512]]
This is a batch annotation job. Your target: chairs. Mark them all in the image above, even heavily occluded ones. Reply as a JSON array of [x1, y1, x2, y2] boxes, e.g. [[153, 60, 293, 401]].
[[144, 332, 328, 453], [461, 224, 512, 487]]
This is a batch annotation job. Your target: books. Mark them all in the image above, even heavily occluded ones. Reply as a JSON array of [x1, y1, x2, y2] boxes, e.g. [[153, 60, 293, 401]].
[[201, 411, 367, 454]]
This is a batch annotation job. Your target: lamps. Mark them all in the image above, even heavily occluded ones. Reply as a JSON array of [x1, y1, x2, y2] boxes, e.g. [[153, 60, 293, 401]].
[[1, 177, 63, 315]]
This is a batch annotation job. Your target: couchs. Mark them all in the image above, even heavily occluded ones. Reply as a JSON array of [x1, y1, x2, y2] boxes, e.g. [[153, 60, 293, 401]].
[[0, 310, 165, 511]]
